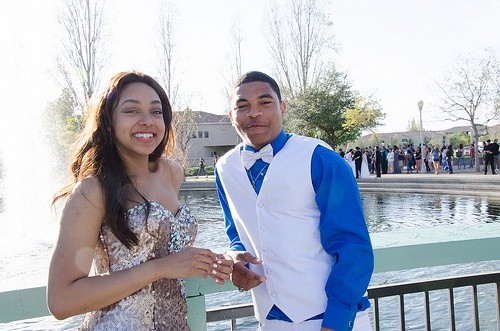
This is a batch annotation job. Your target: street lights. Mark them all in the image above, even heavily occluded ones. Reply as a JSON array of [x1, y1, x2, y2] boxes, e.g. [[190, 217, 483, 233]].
[[317, 130, 321, 140], [417, 99, 428, 173], [73, 105, 82, 136]]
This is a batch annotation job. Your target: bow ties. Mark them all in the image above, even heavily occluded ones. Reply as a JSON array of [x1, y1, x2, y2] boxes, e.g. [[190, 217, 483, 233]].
[[240, 143, 274, 170]]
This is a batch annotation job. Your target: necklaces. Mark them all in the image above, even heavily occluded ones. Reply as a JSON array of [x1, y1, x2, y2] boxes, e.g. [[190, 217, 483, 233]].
[[248, 164, 270, 189]]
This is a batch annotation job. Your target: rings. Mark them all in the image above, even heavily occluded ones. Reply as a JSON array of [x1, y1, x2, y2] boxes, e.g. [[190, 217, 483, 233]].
[[239, 287, 245, 293]]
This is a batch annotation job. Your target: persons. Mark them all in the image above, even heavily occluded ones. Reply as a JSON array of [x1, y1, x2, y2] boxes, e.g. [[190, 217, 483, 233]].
[[214, 71, 374, 331], [338, 139, 500, 178], [46, 71, 234, 331], [195, 155, 220, 179]]
